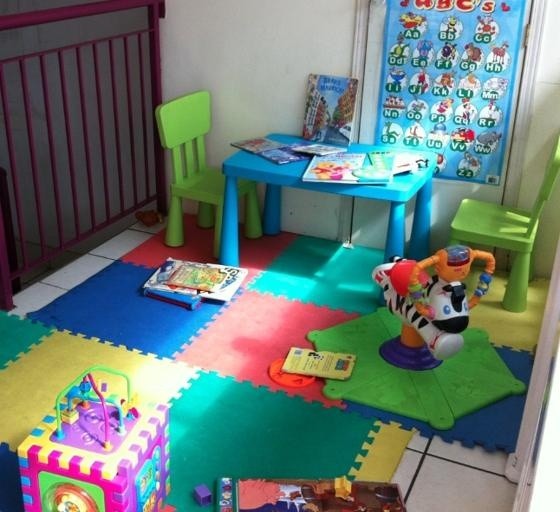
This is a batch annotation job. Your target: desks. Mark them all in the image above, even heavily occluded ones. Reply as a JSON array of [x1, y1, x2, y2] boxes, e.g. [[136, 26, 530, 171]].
[[220, 132, 437, 308]]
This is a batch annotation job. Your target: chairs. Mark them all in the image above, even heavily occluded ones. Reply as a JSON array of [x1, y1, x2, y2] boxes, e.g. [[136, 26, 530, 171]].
[[447, 131, 560, 315], [155, 91, 265, 259]]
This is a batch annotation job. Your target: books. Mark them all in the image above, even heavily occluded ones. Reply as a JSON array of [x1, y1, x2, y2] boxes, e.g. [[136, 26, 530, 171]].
[[140, 256, 250, 310], [283, 346, 357, 380], [214, 474, 405, 512], [229, 72, 430, 185]]
